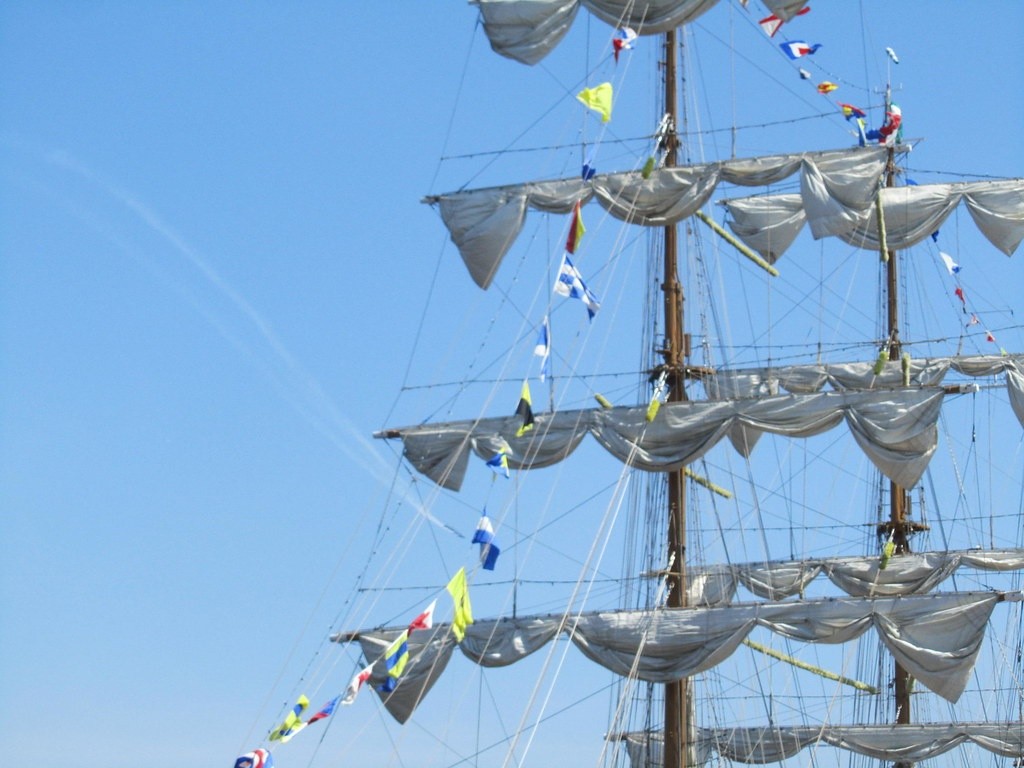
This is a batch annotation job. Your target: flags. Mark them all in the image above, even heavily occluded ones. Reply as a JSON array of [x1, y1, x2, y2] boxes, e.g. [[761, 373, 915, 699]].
[[534, 316, 550, 384], [840, 102, 866, 121], [939, 252, 963, 275], [817, 81, 837, 94], [446, 567, 473, 642], [879, 103, 903, 146], [780, 40, 823, 61], [470, 509, 499, 571], [799, 68, 811, 80], [954, 286, 1007, 356], [906, 179, 918, 185], [758, 6, 810, 38], [932, 230, 938, 242], [576, 82, 612, 122], [234, 629, 409, 768], [564, 200, 587, 253], [614, 27, 638, 64], [865, 130, 886, 140], [408, 599, 435, 637], [485, 446, 510, 479], [515, 382, 535, 436], [554, 255, 601, 323]]
